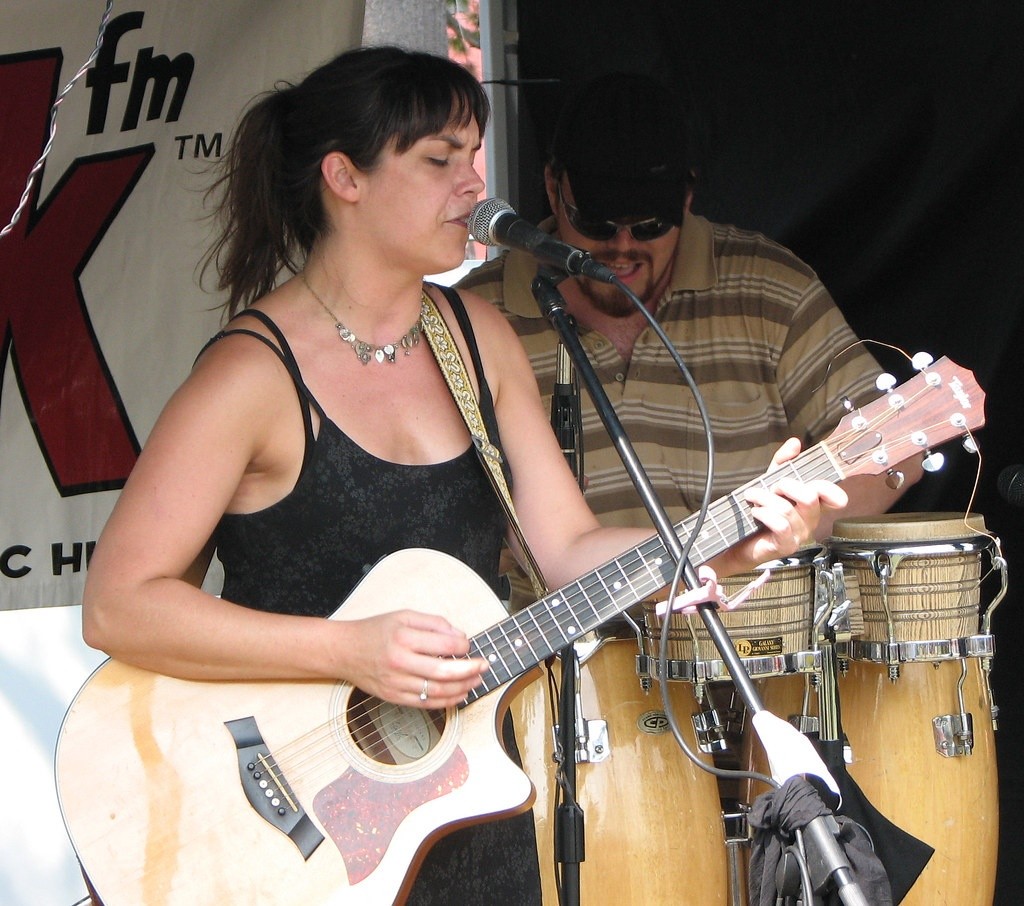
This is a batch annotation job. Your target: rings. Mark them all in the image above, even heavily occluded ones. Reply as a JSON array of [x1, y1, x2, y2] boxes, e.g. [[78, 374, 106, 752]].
[[420, 678, 427, 700]]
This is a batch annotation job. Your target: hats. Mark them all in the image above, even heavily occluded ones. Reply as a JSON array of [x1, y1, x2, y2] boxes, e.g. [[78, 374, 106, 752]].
[[543, 72, 690, 227]]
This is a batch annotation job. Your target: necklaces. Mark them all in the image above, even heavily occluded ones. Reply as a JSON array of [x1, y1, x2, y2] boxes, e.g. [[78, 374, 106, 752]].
[[301, 264, 425, 365]]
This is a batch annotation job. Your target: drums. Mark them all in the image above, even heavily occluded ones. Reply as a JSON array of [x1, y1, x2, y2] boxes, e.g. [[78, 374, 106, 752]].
[[635, 542, 827, 682], [511, 636, 728, 906], [737, 652, 998, 906], [822, 515, 999, 665]]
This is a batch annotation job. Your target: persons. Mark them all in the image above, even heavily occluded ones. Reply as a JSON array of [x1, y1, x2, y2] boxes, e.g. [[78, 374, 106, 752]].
[[82, 45, 849, 906], [447, 80, 925, 617]]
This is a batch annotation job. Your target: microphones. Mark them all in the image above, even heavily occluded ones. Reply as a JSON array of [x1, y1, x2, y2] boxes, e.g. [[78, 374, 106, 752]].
[[997, 465, 1024, 507], [468, 198, 616, 285]]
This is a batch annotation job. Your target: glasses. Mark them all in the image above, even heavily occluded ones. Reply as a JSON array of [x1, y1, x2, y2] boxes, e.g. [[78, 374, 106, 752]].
[[557, 173, 674, 245]]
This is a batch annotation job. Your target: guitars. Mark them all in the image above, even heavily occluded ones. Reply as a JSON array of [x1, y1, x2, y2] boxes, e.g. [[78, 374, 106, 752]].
[[53, 351, 986, 906]]
